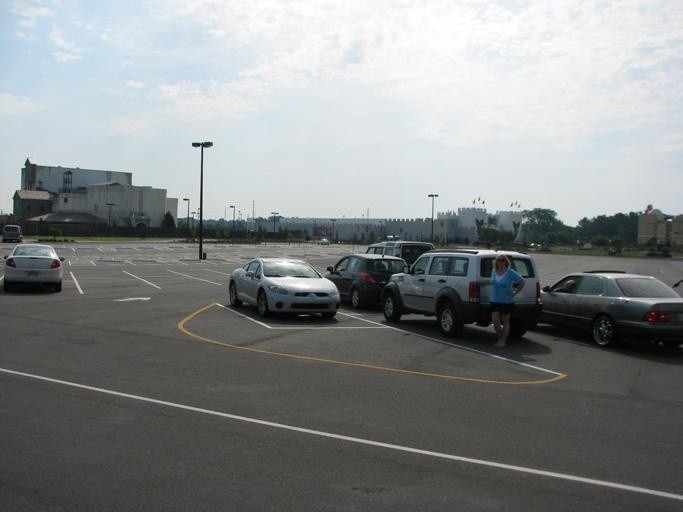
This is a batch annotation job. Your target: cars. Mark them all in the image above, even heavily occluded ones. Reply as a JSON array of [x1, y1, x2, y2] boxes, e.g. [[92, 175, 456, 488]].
[[318, 239, 329, 245], [3, 244, 65, 292]]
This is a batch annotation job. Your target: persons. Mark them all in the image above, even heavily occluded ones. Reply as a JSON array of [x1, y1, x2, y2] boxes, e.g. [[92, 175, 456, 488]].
[[482, 254, 528, 348]]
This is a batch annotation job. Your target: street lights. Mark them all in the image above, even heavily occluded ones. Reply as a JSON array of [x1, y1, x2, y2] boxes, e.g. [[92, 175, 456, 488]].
[[183, 199, 190, 243], [106, 203, 117, 227], [230, 206, 236, 229], [192, 141, 213, 261], [427, 194, 439, 243], [271, 212, 279, 232], [330, 219, 337, 240]]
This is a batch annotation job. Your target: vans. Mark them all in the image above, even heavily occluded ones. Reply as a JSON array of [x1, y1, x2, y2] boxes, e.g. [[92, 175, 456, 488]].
[[2, 225, 23, 243]]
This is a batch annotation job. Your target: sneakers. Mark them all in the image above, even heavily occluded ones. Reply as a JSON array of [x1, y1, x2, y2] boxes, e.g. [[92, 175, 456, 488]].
[[493, 341, 507, 347]]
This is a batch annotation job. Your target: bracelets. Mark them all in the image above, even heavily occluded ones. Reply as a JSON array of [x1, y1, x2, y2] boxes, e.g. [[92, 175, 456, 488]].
[[512, 287, 519, 295]]
